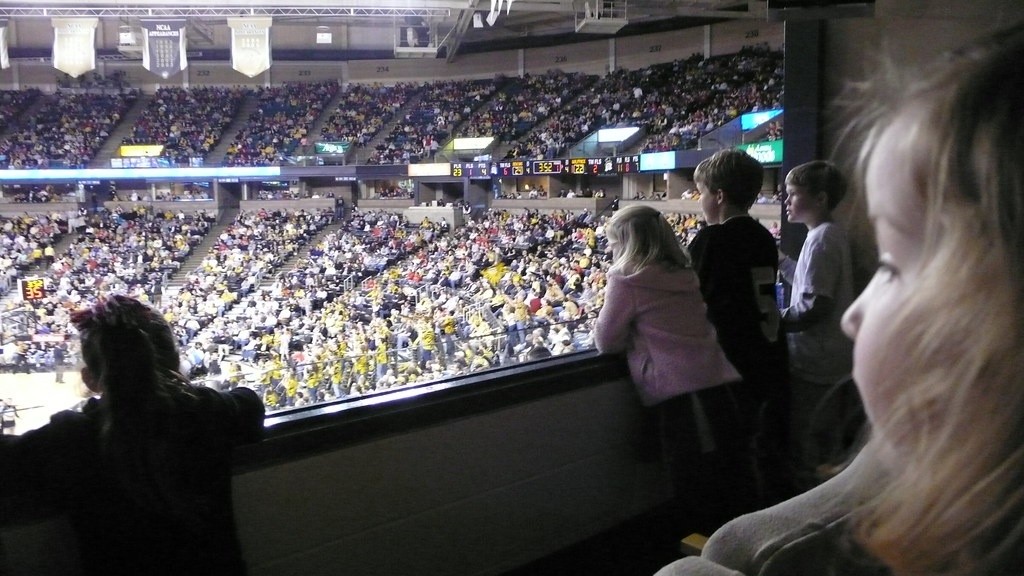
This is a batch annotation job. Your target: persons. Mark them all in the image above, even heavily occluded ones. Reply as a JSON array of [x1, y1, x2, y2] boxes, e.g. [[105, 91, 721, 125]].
[[121, 84, 248, 164], [777, 160, 854, 492], [0, 293, 267, 576], [840, 42, 1024, 576], [0, 183, 782, 409], [456, 39, 784, 161], [222, 79, 338, 165], [595, 204, 743, 524], [320, 73, 505, 164], [686, 147, 790, 508], [0, 69, 136, 171]]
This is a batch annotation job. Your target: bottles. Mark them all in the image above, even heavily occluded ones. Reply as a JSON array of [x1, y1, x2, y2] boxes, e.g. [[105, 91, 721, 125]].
[[774, 269, 792, 309]]
